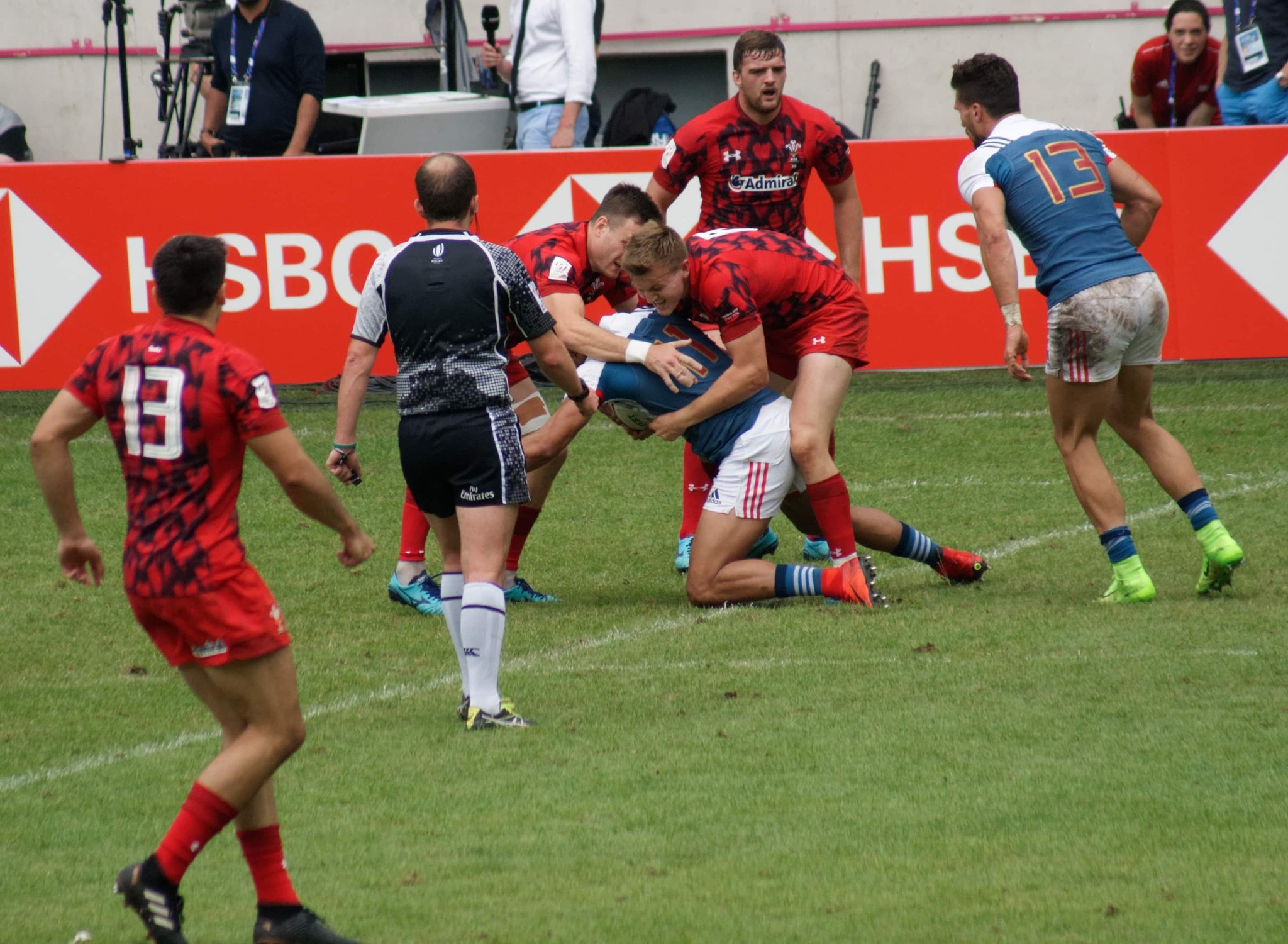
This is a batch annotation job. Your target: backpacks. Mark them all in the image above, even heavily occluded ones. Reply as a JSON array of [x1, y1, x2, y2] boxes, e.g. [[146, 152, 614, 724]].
[[603, 87, 677, 146]]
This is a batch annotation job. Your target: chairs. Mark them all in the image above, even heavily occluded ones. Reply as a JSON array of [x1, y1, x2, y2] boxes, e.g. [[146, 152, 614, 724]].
[[601, 87, 677, 147]]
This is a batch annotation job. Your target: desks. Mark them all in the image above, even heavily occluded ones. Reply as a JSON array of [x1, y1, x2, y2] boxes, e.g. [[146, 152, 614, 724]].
[[322, 90, 511, 155]]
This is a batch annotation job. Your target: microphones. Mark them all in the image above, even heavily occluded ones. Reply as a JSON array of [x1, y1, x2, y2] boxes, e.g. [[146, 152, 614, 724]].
[[482, 6, 500, 78]]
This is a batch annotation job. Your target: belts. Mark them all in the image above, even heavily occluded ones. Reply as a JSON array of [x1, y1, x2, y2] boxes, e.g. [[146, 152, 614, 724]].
[[519, 97, 564, 110]]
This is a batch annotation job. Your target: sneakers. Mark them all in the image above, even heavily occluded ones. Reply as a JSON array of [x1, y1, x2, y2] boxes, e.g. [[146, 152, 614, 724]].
[[503, 576, 565, 603], [465, 701, 538, 731], [842, 555, 890, 609], [933, 545, 991, 584], [253, 908, 357, 944], [387, 569, 444, 618], [745, 526, 779, 558], [1093, 555, 1157, 604], [113, 853, 190, 944], [825, 597, 842, 605], [675, 533, 695, 572], [803, 535, 834, 562], [456, 692, 517, 719], [1194, 519, 1243, 597]]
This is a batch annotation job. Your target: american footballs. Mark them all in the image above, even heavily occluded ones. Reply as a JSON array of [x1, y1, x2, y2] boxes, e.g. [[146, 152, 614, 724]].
[[607, 398, 658, 431]]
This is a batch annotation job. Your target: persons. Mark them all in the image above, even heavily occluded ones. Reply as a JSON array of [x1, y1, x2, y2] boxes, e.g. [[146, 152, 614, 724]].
[[324, 30, 989, 749], [0, 103, 29, 164], [32, 234, 376, 944], [949, 54, 1246, 603], [480, 0, 598, 151], [190, 63, 215, 159], [1213, 0, 1288, 125], [201, 0, 326, 155], [1129, 0, 1222, 131]]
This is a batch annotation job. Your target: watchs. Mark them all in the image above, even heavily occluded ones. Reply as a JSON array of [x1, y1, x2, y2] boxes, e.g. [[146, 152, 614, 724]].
[[200, 129, 216, 137], [567, 378, 590, 402]]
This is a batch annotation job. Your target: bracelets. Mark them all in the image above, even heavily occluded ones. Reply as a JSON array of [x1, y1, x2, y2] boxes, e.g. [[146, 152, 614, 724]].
[[333, 441, 357, 448]]
